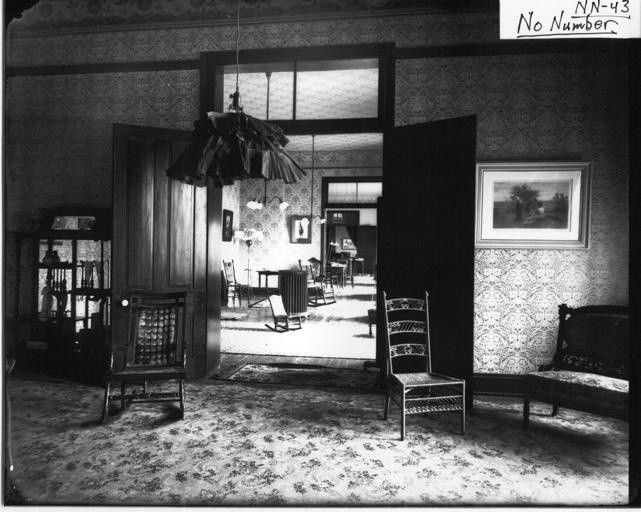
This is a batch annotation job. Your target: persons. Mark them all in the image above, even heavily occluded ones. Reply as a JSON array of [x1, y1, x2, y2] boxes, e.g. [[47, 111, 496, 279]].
[[514, 196, 545, 222]]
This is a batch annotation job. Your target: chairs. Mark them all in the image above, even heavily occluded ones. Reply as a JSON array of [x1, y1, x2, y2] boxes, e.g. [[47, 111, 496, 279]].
[[256, 251, 365, 333], [100, 289, 190, 424], [222, 259, 241, 307], [378, 289, 466, 442]]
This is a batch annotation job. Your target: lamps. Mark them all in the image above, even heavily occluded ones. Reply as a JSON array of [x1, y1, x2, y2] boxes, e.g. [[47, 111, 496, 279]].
[[305, 132, 328, 227], [162, 2, 307, 188], [234, 226, 263, 306], [245, 68, 288, 211]]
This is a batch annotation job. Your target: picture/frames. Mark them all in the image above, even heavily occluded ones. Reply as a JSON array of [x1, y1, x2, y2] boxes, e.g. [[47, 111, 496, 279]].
[[289, 214, 312, 244], [222, 208, 233, 241], [474, 159, 593, 251], [341, 237, 360, 250]]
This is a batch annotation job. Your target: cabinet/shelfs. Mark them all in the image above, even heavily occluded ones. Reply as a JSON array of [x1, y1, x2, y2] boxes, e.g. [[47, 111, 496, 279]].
[[37, 236, 114, 335]]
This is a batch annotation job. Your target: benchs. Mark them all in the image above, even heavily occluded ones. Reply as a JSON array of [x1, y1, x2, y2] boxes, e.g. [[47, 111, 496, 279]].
[[520, 305, 630, 427]]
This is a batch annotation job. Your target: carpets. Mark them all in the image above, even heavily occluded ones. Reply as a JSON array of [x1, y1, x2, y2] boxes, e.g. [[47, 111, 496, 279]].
[[210, 359, 385, 397]]
